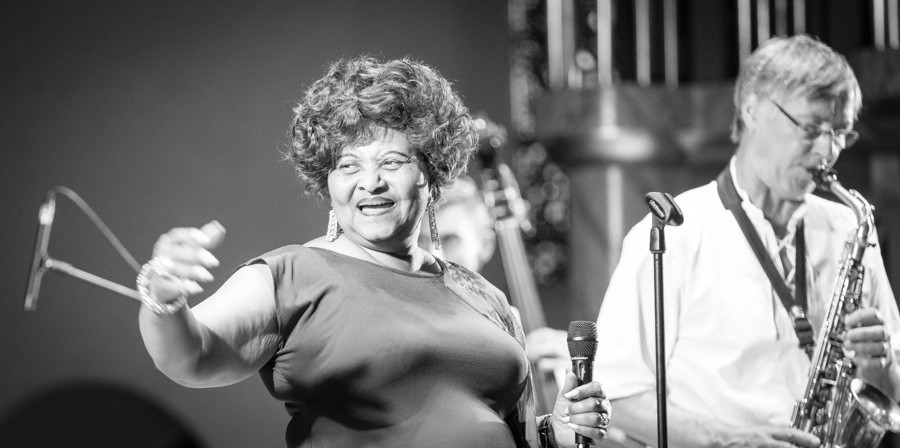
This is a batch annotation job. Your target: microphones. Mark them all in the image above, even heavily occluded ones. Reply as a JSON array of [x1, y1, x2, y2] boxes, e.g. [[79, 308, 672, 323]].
[[566, 321, 599, 448], [24, 189, 56, 310]]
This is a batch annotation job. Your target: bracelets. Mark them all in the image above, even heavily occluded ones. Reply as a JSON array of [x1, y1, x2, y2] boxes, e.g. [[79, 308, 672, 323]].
[[538, 415, 558, 448], [137, 257, 187, 316]]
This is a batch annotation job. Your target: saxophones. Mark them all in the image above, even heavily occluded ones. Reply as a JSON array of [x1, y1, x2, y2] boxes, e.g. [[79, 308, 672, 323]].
[[787, 158, 899, 447]]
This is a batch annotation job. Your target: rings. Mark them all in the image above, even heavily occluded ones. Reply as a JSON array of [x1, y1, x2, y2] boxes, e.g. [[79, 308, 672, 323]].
[[600, 413, 610, 427], [150, 257, 175, 279]]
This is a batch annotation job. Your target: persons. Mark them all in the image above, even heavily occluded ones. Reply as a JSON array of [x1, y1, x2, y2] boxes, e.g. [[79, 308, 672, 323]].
[[140, 57, 613, 448], [592, 37, 900, 448]]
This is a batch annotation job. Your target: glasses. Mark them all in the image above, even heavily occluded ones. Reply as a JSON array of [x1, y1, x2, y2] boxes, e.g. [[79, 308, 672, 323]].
[[770, 99, 859, 150]]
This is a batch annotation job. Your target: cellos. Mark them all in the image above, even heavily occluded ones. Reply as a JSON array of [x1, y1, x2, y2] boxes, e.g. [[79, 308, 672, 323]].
[[467, 111, 564, 413]]
